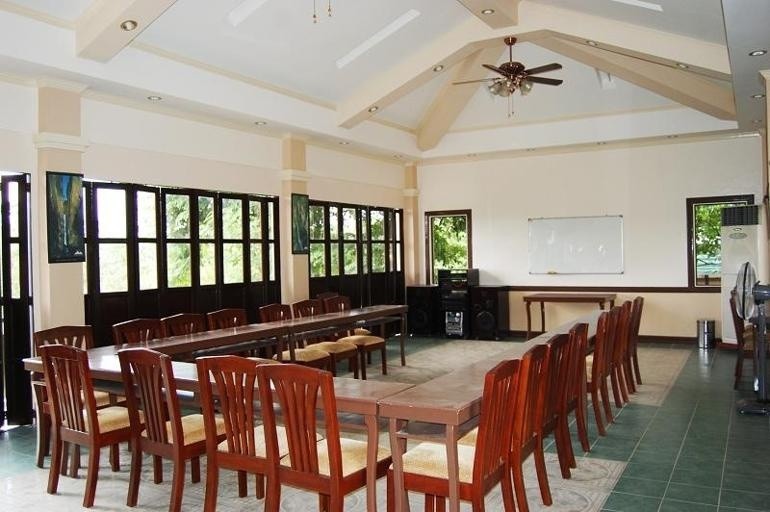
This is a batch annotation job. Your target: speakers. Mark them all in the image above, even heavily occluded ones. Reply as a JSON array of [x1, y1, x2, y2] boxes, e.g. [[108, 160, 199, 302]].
[[472, 284, 510, 340], [406, 283, 441, 339]]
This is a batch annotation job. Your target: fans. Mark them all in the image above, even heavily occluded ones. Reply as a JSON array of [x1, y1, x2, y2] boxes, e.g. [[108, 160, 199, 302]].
[[452, 37, 563, 92], [730, 263, 770, 417]]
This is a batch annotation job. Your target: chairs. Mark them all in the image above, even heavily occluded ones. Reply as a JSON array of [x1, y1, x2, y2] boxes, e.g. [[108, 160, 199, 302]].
[[39, 344, 163, 508], [729, 284, 770, 390], [255, 363, 391, 512], [196, 354, 323, 512], [117, 347, 247, 512]]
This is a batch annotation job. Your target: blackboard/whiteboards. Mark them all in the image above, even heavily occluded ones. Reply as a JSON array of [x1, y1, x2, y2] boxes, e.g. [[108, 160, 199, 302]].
[[527, 214, 624, 274]]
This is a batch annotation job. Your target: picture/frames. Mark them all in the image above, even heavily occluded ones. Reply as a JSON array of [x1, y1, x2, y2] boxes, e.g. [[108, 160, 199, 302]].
[[291, 194, 310, 254], [46, 171, 86, 263]]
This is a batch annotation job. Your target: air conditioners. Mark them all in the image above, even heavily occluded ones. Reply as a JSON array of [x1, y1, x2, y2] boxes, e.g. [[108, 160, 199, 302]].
[[720, 203, 767, 346]]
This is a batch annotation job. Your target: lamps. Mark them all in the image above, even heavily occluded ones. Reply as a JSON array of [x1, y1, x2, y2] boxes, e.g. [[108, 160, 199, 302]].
[[488, 80, 533, 121]]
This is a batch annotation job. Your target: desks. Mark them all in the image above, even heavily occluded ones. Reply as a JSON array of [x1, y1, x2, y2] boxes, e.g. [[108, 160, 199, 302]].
[[23, 303, 409, 366], [23, 303, 416, 511], [376, 310, 617, 511], [523, 293, 617, 341]]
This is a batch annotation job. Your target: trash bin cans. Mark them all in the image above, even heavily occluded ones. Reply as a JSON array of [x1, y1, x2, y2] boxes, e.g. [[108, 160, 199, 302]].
[[698, 319, 715, 349]]
